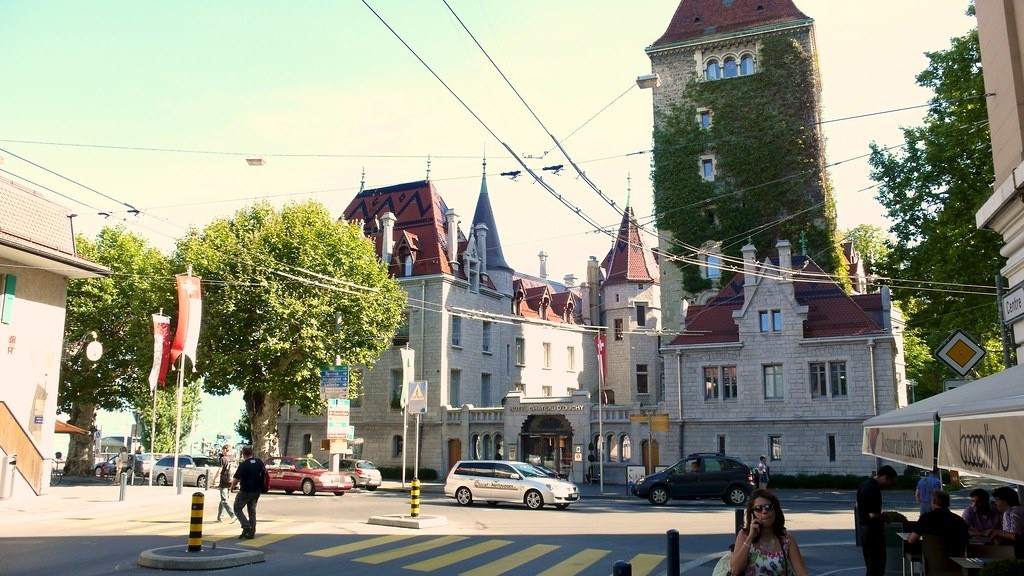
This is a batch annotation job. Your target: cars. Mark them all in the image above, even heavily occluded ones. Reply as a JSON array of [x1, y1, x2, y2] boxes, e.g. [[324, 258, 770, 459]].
[[261, 457, 354, 496], [93, 452, 158, 477], [153, 454, 222, 488], [534, 464, 568, 481], [321, 459, 382, 491]]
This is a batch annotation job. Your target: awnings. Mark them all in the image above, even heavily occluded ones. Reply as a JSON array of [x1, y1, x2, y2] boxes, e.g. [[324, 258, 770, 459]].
[[862, 362, 1024, 486]]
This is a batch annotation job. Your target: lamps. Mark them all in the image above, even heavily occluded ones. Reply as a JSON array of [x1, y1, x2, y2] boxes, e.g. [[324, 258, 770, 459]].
[[635, 74, 659, 89]]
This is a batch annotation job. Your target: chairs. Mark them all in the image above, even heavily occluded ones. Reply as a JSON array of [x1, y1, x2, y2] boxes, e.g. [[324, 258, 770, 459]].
[[901, 521, 1016, 576]]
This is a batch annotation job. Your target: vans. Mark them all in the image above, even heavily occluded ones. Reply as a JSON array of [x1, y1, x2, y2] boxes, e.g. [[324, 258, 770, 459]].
[[444, 460, 580, 510]]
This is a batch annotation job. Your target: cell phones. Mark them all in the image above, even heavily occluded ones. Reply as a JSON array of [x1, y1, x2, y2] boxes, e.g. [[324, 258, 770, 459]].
[[752, 513, 758, 525]]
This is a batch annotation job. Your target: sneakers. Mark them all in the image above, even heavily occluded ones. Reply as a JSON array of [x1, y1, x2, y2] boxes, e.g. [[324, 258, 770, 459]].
[[239, 527, 256, 539], [229, 515, 238, 524], [214, 518, 224, 522]]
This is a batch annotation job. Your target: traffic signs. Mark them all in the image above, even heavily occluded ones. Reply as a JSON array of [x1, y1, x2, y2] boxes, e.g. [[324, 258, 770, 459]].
[[318, 365, 349, 398]]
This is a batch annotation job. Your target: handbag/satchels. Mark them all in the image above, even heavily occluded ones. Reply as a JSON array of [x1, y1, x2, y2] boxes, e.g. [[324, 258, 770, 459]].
[[712, 544, 745, 576]]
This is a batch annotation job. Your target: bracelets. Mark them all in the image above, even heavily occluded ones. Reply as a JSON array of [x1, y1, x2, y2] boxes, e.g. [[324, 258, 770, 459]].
[[744, 541, 751, 549]]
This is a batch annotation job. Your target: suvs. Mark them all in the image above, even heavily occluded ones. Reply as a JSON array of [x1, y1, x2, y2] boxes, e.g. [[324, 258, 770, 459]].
[[631, 453, 755, 506]]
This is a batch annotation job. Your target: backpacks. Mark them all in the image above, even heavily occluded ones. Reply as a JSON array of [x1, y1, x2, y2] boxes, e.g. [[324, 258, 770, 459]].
[[220, 456, 239, 484], [256, 459, 270, 495]]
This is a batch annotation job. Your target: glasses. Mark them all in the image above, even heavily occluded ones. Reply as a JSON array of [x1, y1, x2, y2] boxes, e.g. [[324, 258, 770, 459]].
[[751, 503, 776, 513]]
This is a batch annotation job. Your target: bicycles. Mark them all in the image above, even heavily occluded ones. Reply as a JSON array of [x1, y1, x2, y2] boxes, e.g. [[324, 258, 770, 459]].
[[106, 467, 145, 485], [51, 470, 61, 485]]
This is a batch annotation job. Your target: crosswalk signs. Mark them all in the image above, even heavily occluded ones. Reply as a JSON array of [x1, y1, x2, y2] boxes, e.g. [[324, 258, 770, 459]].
[[408, 381, 428, 413]]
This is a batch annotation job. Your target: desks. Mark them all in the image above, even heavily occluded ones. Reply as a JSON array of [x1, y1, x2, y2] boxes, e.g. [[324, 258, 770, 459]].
[[895, 532, 1014, 576]]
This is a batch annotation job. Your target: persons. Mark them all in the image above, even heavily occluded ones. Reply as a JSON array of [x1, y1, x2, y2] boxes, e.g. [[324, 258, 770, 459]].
[[758, 455, 768, 490], [230, 445, 266, 539], [915, 466, 940, 515], [730, 489, 808, 576], [212, 445, 239, 524], [691, 462, 698, 470], [908, 491, 969, 576], [136, 445, 143, 454], [857, 465, 897, 576], [114, 457, 122, 485], [120, 446, 128, 471], [962, 489, 1002, 545], [985, 486, 1024, 560]]
